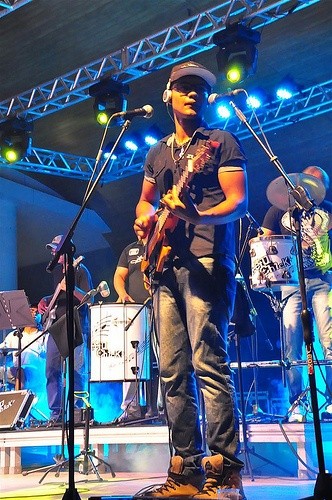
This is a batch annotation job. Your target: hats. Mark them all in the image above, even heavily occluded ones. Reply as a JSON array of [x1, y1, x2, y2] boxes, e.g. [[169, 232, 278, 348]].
[[167, 61, 217, 90], [44, 235, 76, 253]]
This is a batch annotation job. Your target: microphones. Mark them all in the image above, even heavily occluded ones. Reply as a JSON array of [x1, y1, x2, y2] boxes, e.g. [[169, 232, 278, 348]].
[[83, 282, 110, 297], [245, 210, 264, 235], [114, 105, 154, 119], [208, 89, 245, 104]]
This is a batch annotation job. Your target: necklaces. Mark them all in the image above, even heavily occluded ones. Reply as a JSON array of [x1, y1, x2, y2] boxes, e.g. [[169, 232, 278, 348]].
[[171, 137, 191, 165]]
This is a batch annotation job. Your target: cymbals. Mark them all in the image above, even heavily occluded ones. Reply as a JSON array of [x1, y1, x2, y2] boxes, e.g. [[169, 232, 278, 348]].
[[266, 172, 326, 212], [0, 348, 20, 353]]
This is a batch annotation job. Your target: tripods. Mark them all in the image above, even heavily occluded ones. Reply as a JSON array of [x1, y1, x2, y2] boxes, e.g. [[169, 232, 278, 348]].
[[227, 277, 295, 483]]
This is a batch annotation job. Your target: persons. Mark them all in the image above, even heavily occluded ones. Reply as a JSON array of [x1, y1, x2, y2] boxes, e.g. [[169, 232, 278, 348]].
[[38, 235, 95, 426], [113, 239, 166, 421], [0, 302, 49, 390], [135, 60, 248, 500], [257, 165, 332, 423]]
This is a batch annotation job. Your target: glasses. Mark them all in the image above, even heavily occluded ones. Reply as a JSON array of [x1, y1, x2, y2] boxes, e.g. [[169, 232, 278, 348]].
[[171, 83, 207, 97]]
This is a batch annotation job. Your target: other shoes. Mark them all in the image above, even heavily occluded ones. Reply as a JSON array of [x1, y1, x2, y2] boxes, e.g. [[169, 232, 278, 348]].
[[115, 406, 141, 423], [320, 404, 332, 422], [145, 407, 154, 419], [39, 418, 62, 426], [288, 406, 308, 423]]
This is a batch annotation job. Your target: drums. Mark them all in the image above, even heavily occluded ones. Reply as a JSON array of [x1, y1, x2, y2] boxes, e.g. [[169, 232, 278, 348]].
[[250, 235, 300, 291], [91, 303, 149, 383]]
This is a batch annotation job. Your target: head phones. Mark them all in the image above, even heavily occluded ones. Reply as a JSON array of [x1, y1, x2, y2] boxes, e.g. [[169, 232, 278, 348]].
[[163, 77, 172, 103]]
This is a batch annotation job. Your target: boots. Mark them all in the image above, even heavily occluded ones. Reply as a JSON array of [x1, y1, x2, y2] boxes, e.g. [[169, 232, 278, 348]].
[[188, 452, 247, 500], [132, 451, 206, 500]]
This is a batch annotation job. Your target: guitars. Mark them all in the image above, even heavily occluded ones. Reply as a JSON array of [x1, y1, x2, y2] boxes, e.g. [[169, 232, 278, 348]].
[[41, 255, 86, 345], [141, 138, 223, 289]]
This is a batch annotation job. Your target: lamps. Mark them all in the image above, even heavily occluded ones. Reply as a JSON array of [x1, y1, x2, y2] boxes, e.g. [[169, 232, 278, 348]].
[[0, 118, 34, 162], [246, 88, 267, 108], [274, 74, 296, 100], [214, 24, 262, 85], [125, 133, 141, 150], [103, 142, 119, 159], [144, 125, 159, 145], [88, 78, 131, 128]]
